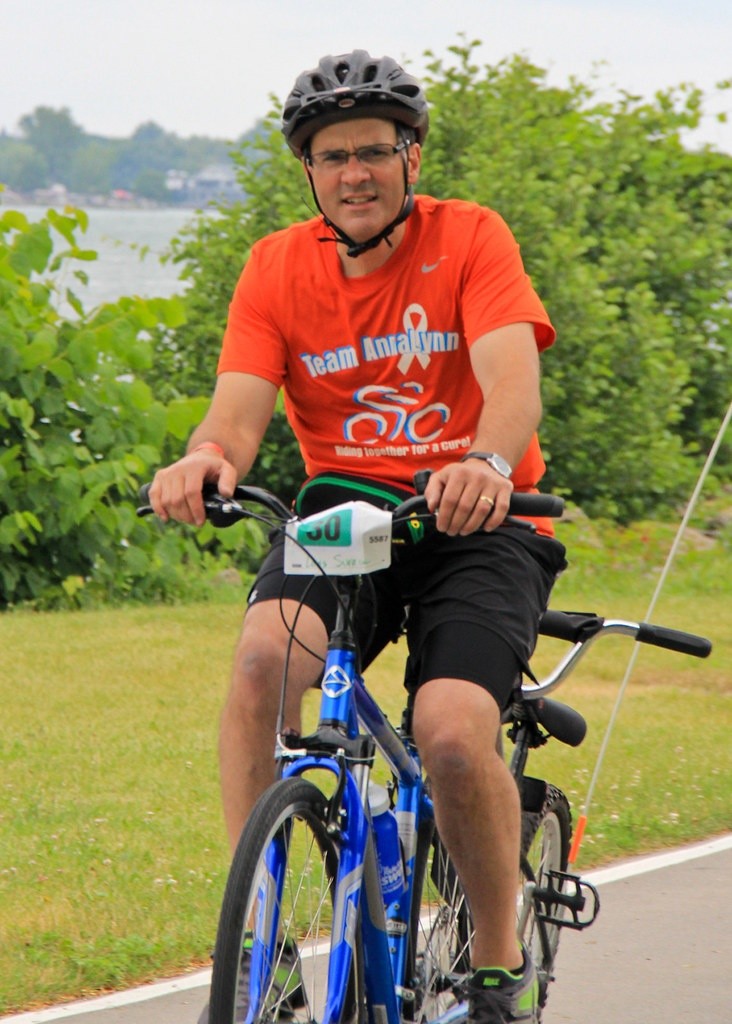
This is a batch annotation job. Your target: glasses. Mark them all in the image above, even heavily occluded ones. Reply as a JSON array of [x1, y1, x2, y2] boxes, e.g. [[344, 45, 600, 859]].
[[306, 140, 410, 175]]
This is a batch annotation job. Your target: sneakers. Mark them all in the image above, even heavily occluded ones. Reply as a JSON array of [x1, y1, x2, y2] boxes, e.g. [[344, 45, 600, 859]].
[[197, 932, 308, 1022], [468, 941, 539, 1024]]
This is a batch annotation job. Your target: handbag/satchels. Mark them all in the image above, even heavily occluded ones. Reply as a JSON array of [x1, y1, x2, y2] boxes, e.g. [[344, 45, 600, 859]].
[[296, 470, 436, 551]]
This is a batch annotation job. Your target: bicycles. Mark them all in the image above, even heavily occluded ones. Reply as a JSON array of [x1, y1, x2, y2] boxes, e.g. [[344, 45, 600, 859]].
[[453, 588, 712, 1024], [128, 470, 573, 1024]]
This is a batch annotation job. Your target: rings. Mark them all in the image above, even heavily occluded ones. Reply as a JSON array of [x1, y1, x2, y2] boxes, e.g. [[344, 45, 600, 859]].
[[481, 496, 494, 506]]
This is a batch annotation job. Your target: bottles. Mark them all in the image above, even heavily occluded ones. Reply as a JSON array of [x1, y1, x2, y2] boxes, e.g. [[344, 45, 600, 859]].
[[362, 779, 408, 906]]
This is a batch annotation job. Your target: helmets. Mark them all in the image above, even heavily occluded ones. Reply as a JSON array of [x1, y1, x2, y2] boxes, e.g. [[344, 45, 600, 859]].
[[281, 50, 429, 159]]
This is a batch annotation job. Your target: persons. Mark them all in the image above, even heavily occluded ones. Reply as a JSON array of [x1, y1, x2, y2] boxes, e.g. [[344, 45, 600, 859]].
[[147, 49, 570, 1024]]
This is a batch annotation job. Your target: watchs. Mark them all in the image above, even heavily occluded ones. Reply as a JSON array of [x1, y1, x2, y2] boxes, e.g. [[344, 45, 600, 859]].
[[460, 451, 513, 479]]
